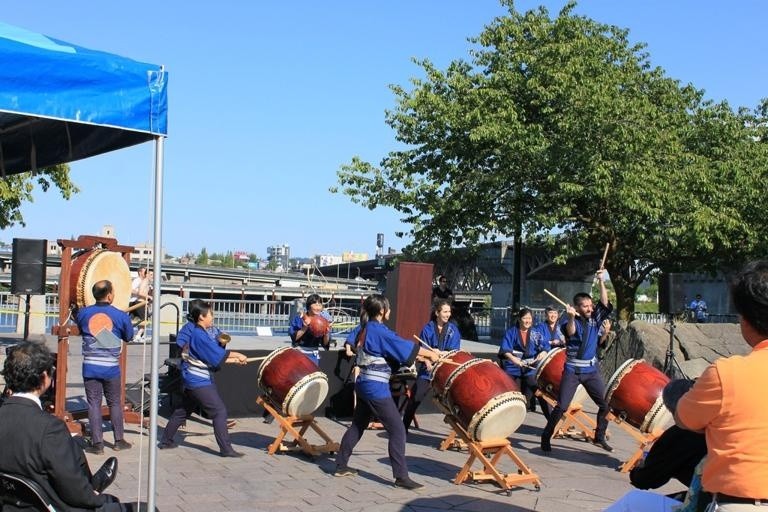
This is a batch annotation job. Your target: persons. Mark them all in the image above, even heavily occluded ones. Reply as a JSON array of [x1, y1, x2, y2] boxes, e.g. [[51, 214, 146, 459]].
[[263, 294, 332, 424], [91, 457, 117, 493], [403, 298, 460, 433], [129, 267, 145, 315], [630, 380, 708, 503], [0, 339, 159, 512], [177, 300, 236, 430], [537, 306, 566, 350], [76, 280, 133, 454], [690, 294, 707, 324], [431, 277, 455, 301], [158, 303, 247, 457], [346, 325, 362, 411], [133, 270, 153, 344], [675, 258, 768, 512], [332, 294, 437, 490], [541, 271, 614, 452], [498, 308, 550, 412], [598, 319, 611, 349]]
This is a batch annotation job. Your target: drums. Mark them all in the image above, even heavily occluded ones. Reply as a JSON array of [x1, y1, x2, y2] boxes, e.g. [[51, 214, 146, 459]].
[[58, 249, 132, 325], [428, 349, 477, 399], [442, 358, 527, 444], [533, 347, 590, 408], [257, 347, 328, 417], [603, 358, 673, 433]]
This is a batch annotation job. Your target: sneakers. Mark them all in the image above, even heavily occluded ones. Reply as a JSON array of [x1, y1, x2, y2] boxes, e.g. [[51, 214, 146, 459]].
[[592, 438, 616, 454], [113, 440, 132, 450], [334, 469, 359, 478], [85, 443, 105, 455], [263, 417, 275, 424], [541, 443, 553, 452], [221, 450, 248, 457], [158, 442, 179, 450], [226, 419, 239, 429], [393, 477, 428, 492]]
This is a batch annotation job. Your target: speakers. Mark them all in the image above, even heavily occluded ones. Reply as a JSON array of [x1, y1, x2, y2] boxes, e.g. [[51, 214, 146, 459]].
[[10, 239, 44, 296], [659, 275, 685, 317]]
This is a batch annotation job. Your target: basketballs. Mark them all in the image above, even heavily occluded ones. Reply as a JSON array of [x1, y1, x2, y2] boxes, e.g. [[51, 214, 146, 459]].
[[309, 317, 328, 336]]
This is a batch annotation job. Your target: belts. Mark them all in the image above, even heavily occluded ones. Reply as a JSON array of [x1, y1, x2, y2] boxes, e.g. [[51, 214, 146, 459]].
[[704, 492, 754, 507]]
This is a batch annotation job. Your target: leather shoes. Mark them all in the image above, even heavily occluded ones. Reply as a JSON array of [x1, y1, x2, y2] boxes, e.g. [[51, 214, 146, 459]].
[[94, 456, 118, 487]]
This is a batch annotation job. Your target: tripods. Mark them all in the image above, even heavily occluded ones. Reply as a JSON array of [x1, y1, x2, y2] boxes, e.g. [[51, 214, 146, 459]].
[[599, 321, 628, 371], [663, 318, 687, 387]]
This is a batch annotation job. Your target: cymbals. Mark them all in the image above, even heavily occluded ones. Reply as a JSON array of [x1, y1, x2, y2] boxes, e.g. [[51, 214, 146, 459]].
[[217, 333, 231, 344]]
